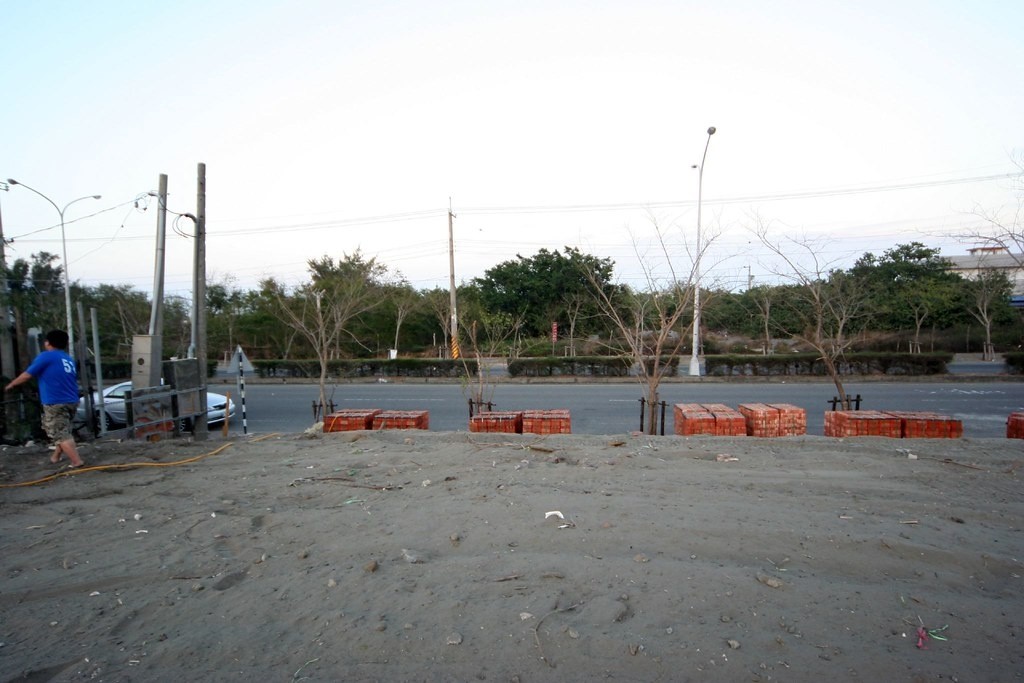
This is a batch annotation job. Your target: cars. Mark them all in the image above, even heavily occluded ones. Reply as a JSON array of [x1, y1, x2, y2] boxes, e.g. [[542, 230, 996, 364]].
[[76, 381, 236, 433]]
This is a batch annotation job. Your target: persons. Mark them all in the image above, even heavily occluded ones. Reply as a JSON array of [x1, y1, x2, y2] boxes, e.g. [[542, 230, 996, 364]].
[[3, 330, 85, 468]]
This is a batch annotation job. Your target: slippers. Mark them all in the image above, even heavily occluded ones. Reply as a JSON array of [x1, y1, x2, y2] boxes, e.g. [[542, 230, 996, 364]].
[[68, 464, 84, 468]]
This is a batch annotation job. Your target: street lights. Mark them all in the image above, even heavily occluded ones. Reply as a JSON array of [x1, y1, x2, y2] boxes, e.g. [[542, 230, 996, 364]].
[[6, 178, 101, 359], [690, 127, 718, 376]]
